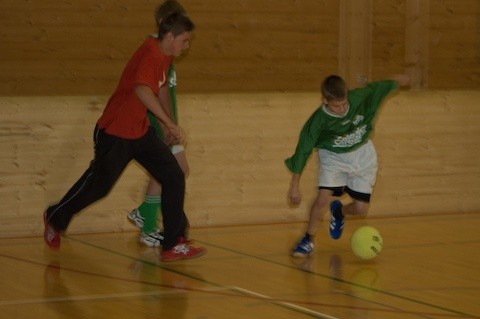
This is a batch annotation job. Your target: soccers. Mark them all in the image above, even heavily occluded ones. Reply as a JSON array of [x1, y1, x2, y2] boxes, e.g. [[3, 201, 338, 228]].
[[350, 226, 384, 259]]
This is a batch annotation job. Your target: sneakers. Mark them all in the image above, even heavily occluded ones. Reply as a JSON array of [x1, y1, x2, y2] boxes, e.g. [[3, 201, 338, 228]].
[[329, 199, 345, 239], [127, 208, 145, 229], [159, 239, 207, 262], [43, 212, 61, 250], [138, 228, 164, 247], [291, 232, 315, 258]]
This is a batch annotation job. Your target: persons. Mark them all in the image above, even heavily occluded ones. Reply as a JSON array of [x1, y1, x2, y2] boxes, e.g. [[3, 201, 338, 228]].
[[284, 74, 412, 258], [43, 0, 207, 260]]
